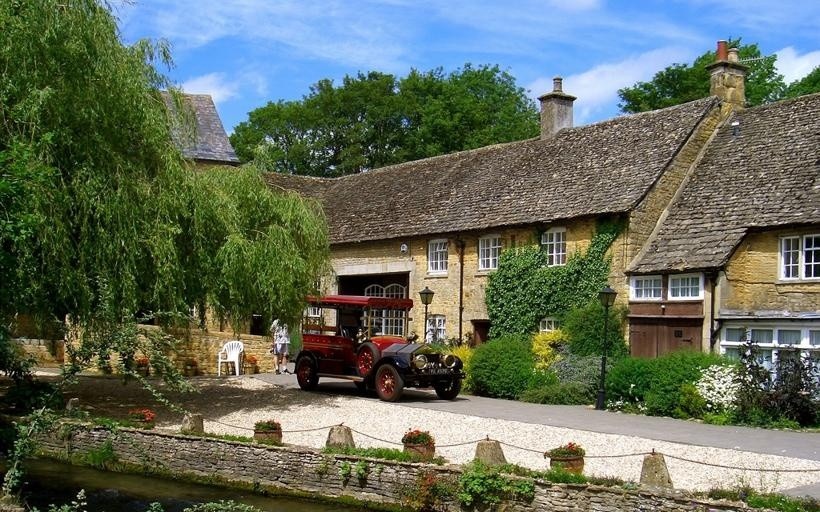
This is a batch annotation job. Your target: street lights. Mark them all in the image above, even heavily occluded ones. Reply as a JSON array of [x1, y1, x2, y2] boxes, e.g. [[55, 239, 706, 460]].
[[418, 286, 435, 344], [593, 285, 619, 411]]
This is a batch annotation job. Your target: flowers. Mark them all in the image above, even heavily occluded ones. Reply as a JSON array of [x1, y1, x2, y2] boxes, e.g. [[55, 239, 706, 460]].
[[243, 356, 258, 365], [254, 419, 283, 437], [400, 426, 438, 448], [127, 405, 158, 421], [136, 355, 197, 378], [543, 442, 586, 461]]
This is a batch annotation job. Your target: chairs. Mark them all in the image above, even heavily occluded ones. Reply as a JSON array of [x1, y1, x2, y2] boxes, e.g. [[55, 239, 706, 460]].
[[218, 341, 245, 377]]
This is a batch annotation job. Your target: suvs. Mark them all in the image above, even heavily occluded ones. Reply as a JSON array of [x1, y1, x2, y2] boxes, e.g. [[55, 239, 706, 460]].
[[293, 294, 467, 402]]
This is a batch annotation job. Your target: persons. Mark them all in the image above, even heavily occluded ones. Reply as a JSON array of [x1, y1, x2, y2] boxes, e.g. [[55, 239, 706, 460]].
[[270, 317, 291, 375]]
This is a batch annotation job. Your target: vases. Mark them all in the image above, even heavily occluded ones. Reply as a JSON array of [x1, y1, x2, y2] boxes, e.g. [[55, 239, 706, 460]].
[[253, 429, 283, 445], [548, 454, 585, 475], [130, 420, 156, 432], [244, 363, 255, 375], [403, 442, 437, 462]]
